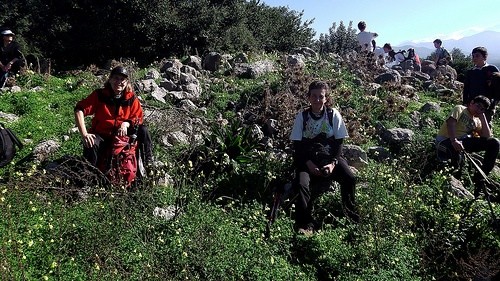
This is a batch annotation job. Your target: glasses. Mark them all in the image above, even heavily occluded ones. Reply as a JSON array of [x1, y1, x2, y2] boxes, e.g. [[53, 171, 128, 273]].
[[111, 77, 128, 82]]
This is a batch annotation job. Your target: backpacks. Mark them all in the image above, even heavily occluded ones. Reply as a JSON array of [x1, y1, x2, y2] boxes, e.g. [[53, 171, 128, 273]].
[[439, 47, 452, 64], [112, 135, 139, 183]]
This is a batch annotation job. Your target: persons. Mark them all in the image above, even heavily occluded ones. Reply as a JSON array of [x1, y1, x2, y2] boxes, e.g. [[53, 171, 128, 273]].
[[289, 79, 364, 229], [0, 29, 28, 87], [73, 66, 159, 192], [406, 48, 421, 65], [357, 21, 379, 66], [382, 43, 399, 67], [462, 45, 500, 122], [433, 39, 448, 67], [435, 94, 500, 180]]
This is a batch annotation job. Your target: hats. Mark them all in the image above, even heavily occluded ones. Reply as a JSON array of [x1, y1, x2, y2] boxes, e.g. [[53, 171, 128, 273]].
[[111, 67, 128, 77]]
